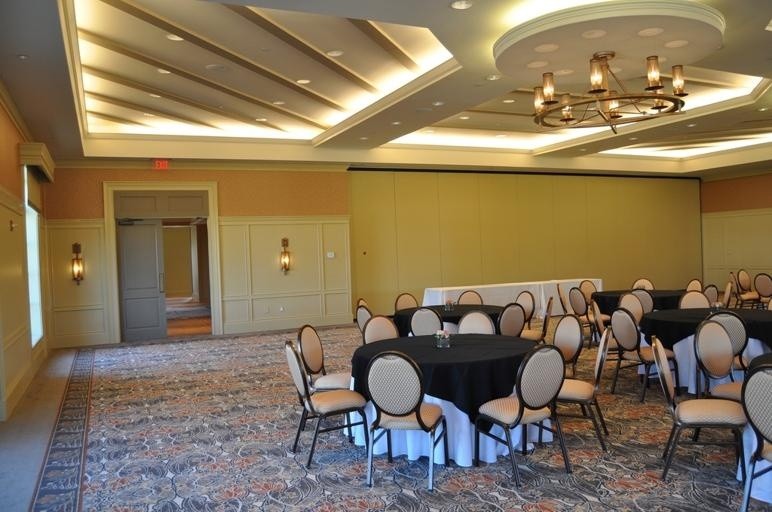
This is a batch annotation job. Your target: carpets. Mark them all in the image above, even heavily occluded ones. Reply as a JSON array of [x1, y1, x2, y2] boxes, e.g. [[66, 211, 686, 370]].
[[28, 323, 771, 512]]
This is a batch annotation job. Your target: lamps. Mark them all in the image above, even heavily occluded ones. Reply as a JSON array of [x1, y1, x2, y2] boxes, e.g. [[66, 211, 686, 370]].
[[280, 237, 290, 273], [533, 51, 688, 134], [72, 242, 83, 285]]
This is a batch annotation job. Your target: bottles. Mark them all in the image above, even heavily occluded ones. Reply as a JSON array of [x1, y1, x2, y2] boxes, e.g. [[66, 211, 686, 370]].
[[434, 329, 450, 348], [710, 302, 719, 315], [443, 299, 455, 311]]
